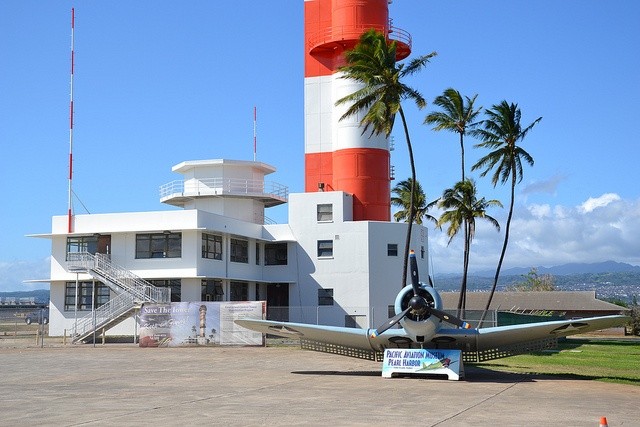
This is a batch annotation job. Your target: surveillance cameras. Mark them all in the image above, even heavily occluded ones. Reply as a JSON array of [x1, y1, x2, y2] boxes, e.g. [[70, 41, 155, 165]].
[[344, 194, 349, 197]]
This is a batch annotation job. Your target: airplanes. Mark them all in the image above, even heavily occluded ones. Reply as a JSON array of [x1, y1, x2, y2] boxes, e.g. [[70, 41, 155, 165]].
[[232, 250, 632, 362]]
